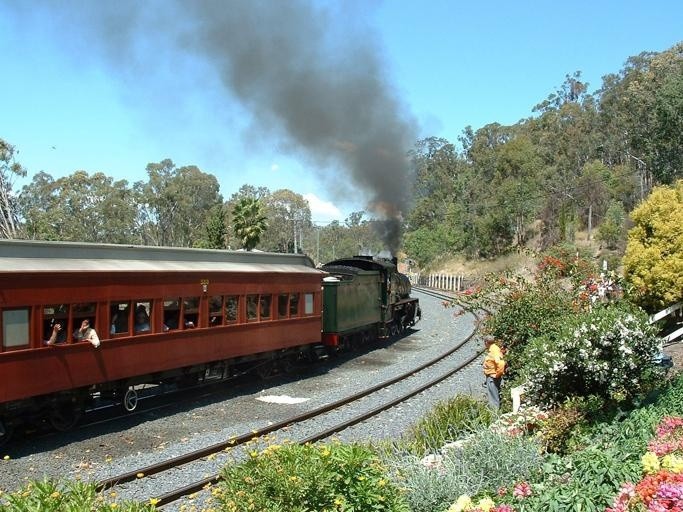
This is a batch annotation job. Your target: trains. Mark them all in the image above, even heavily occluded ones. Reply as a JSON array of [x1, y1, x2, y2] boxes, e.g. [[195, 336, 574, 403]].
[[0, 238, 422, 445]]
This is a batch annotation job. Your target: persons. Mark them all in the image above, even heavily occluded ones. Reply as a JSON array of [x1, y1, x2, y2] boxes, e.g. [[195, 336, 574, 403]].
[[42, 294, 287, 348], [482, 335, 505, 412]]
